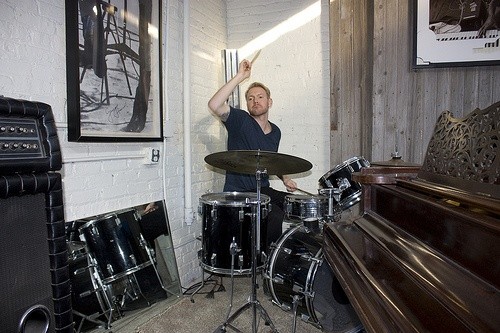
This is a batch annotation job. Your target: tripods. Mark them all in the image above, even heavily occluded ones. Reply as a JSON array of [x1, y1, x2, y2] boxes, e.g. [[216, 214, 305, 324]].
[[204, 149, 313, 333]]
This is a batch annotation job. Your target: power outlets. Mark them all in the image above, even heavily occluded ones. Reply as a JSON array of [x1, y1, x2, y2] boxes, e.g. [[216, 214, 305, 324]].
[[143, 148, 160, 164]]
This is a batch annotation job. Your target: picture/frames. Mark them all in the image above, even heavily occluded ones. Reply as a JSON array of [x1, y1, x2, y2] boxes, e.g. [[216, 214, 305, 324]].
[[65, 0, 164, 143], [408, 0, 500, 71]]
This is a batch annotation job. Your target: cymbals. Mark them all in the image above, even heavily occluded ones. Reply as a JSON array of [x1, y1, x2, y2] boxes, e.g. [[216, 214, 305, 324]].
[[205, 149, 313, 176]]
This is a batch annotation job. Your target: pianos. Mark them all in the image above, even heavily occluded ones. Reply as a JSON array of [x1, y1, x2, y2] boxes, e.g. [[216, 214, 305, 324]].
[[322, 165, 500, 333]]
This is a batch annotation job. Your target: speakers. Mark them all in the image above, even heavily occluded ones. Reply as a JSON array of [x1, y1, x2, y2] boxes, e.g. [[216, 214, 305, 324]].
[[0, 172, 75, 333]]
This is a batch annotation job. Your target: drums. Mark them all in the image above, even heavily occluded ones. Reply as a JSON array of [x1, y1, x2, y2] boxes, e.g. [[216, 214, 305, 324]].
[[318, 156, 372, 211], [265, 217, 364, 333], [284, 194, 324, 220], [200, 191, 273, 277], [78, 208, 154, 285]]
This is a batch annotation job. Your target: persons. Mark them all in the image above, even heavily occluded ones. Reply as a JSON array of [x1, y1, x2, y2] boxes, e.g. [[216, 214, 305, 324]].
[[209, 58, 296, 253], [478, 0, 500, 39]]
